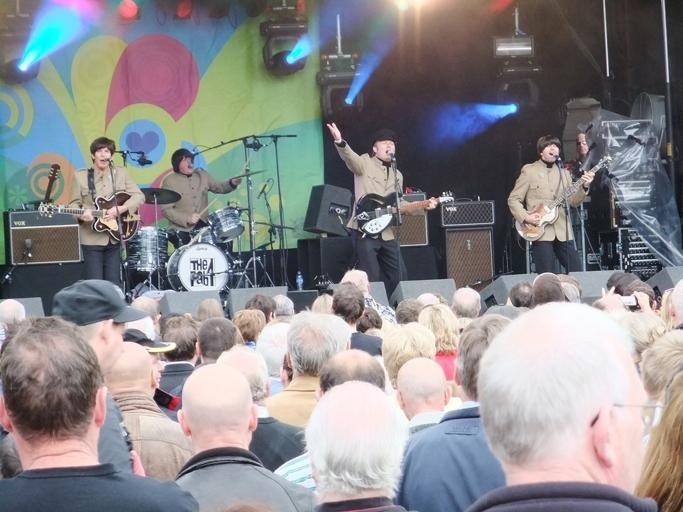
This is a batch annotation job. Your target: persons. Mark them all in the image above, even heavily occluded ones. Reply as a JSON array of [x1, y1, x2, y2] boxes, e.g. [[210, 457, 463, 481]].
[[569, 133, 599, 178], [158, 145, 243, 239], [325, 117, 439, 314], [65, 136, 145, 288], [504, 133, 595, 276], [0, 266, 681, 510]]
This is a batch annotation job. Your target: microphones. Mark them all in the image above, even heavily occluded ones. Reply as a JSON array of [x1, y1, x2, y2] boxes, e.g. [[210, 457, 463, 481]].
[[388, 152, 396, 163], [554, 154, 562, 162], [103, 156, 114, 165]]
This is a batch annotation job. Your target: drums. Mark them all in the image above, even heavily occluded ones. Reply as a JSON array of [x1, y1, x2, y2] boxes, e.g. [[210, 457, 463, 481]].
[[168, 243, 230, 291], [207, 209, 243, 241], [126, 227, 168, 271]]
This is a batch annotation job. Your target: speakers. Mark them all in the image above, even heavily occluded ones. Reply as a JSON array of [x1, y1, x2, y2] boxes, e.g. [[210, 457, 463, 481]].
[[3, 210, 84, 265], [644, 266, 683, 309], [0, 297, 46, 319], [0, 263, 81, 318], [226, 286, 289, 320], [569, 270, 625, 308], [158, 292, 224, 331], [478, 272, 539, 314], [288, 184, 496, 318]]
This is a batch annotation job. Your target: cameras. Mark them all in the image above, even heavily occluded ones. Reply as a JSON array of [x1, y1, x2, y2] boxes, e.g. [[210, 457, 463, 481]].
[[622, 296, 638, 308]]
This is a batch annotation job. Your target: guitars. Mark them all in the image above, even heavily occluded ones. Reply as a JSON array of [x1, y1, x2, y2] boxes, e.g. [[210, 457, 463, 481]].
[[37, 192, 138, 239], [515, 158, 615, 241], [357, 193, 454, 235]]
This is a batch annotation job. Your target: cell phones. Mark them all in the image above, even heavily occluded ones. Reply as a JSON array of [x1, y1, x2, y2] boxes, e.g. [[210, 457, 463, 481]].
[[153, 389, 180, 410]]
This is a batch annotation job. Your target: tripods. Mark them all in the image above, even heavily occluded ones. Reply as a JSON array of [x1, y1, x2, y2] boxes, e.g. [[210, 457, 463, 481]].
[[136, 134, 294, 293]]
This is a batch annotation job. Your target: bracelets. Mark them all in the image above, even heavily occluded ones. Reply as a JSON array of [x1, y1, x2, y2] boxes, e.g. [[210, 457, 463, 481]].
[[333, 137, 341, 144]]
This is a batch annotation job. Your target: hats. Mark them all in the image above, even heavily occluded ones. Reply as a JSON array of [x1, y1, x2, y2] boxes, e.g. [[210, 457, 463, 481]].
[[53, 279, 149, 326], [123, 329, 176, 353]]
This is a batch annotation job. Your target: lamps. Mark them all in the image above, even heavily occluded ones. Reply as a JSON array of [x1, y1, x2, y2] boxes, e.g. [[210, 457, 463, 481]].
[[492, 65, 543, 118], [0, 22, 47, 82], [315, 64, 365, 122], [258, 18, 308, 77]]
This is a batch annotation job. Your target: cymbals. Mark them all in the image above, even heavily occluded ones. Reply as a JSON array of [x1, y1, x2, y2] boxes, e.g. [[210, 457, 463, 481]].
[[233, 169, 265, 179], [138, 188, 181, 205]]
